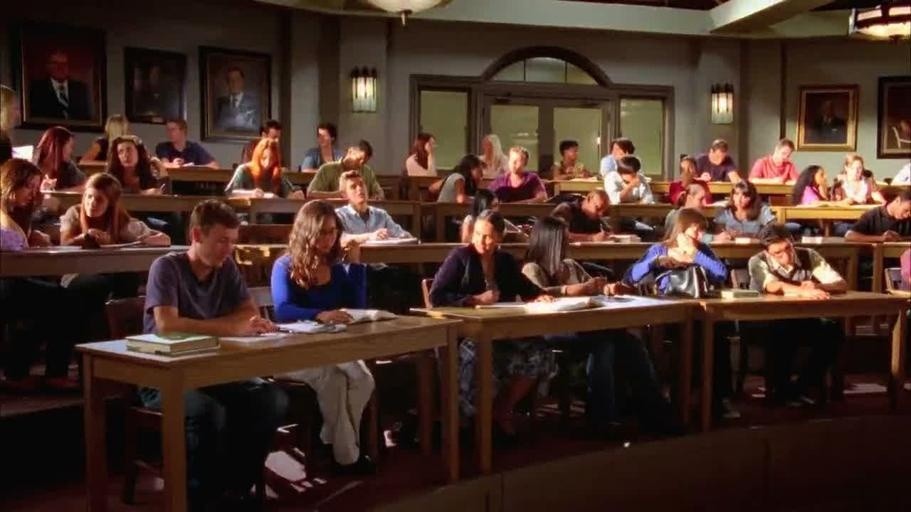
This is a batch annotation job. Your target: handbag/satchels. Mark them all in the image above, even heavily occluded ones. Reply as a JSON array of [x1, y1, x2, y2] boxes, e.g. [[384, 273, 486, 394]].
[[656, 262, 719, 298]]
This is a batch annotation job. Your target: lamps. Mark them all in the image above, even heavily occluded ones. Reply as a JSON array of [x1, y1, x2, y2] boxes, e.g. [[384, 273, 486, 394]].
[[710, 81, 735, 126], [350, 67, 379, 114], [848, 0, 911, 45], [368, 0, 446, 26]]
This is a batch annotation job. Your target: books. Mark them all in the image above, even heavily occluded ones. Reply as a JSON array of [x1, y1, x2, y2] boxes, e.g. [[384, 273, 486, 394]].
[[125, 336, 221, 358], [335, 308, 398, 324], [708, 287, 757, 300], [525, 297, 605, 313]]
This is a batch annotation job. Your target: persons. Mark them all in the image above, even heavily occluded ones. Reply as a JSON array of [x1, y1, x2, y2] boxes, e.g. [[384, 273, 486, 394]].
[[30, 47, 90, 121], [479, 134, 509, 178], [59, 173, 171, 339], [659, 183, 731, 242], [156, 117, 221, 169], [714, 181, 778, 238], [1, 84, 22, 164], [549, 140, 600, 182], [308, 138, 385, 200], [748, 222, 848, 410], [694, 138, 744, 182], [460, 188, 529, 242], [810, 97, 848, 144], [301, 122, 344, 174], [623, 206, 741, 418], [33, 125, 85, 188], [791, 165, 830, 205], [549, 189, 614, 243], [406, 133, 438, 176], [601, 136, 654, 205], [241, 120, 282, 163], [487, 146, 548, 205], [669, 156, 713, 206], [834, 153, 887, 207], [103, 134, 169, 195], [217, 67, 261, 134], [2, 158, 67, 311], [334, 170, 412, 243], [271, 199, 374, 475], [138, 63, 177, 117], [141, 199, 280, 501], [521, 216, 668, 437], [429, 209, 556, 444], [223, 137, 305, 198], [428, 153, 490, 204], [79, 114, 134, 167], [843, 185, 911, 245], [748, 138, 800, 185]]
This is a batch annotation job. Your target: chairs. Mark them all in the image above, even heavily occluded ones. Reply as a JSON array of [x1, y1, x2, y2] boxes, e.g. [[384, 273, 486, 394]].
[[421, 277, 543, 442], [106, 295, 269, 505], [548, 256, 621, 421], [246, 284, 383, 480], [732, 266, 833, 408]]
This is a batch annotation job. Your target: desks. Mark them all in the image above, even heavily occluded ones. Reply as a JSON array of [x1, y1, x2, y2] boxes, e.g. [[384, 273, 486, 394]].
[[36, 191, 277, 219], [603, 201, 731, 236], [566, 237, 814, 263], [339, 239, 558, 273], [281, 172, 404, 201], [653, 287, 908, 434], [74, 159, 108, 175], [411, 172, 562, 203], [165, 167, 237, 192], [425, 201, 569, 242], [1, 242, 264, 397], [73, 313, 465, 512], [777, 206, 884, 229], [410, 292, 702, 473], [555, 176, 809, 201], [249, 195, 422, 238]]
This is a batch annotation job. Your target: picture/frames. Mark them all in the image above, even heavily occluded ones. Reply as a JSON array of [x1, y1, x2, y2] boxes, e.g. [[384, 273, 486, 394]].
[[124, 45, 187, 126], [794, 82, 859, 152], [197, 44, 273, 147], [12, 22, 108, 134], [874, 75, 911, 161]]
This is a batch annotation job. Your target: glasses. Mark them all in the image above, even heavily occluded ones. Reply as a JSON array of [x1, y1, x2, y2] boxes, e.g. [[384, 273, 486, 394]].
[[322, 227, 339, 236]]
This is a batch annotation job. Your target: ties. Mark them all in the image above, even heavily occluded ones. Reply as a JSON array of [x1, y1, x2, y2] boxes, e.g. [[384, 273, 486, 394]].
[[59, 84, 71, 119]]
